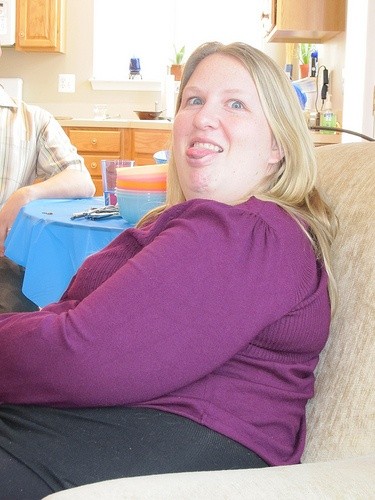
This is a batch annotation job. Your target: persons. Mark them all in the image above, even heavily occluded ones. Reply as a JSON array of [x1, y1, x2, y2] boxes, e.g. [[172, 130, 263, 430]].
[[0, 83, 96, 313], [0, 41, 338, 500]]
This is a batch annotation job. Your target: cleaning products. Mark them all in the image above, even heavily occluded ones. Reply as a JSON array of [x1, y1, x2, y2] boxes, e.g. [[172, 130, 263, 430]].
[[320, 93, 337, 134]]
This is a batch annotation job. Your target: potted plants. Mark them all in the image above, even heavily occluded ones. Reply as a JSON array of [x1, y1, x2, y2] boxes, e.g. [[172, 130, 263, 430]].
[[170, 43, 186, 81], [297, 43, 313, 79]]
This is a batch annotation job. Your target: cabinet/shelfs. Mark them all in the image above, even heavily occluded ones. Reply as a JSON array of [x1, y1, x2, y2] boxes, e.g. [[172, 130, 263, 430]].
[[131, 130, 173, 167], [63, 127, 124, 195], [15, 0, 65, 54], [260, 0, 345, 43]]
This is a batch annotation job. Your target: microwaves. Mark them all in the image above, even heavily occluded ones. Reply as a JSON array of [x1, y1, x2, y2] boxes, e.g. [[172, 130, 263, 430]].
[[0, 0, 16, 48]]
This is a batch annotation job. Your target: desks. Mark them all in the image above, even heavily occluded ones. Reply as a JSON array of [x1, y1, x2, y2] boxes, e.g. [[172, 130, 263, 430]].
[[5, 197, 137, 312]]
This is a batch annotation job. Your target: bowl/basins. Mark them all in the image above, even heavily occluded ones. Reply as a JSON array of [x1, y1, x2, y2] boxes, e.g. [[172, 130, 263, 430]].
[[132, 110, 164, 120], [114, 165, 169, 225], [153, 150, 168, 164]]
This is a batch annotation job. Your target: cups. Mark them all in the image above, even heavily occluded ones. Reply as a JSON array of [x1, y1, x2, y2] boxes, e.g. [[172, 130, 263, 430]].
[[101, 159, 136, 209]]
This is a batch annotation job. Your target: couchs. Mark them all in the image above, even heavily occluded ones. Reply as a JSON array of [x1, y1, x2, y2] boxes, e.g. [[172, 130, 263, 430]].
[[41, 140, 375, 500]]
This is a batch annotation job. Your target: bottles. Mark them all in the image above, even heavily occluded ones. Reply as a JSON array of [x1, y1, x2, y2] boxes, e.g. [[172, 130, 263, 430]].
[[321, 107, 337, 135]]
